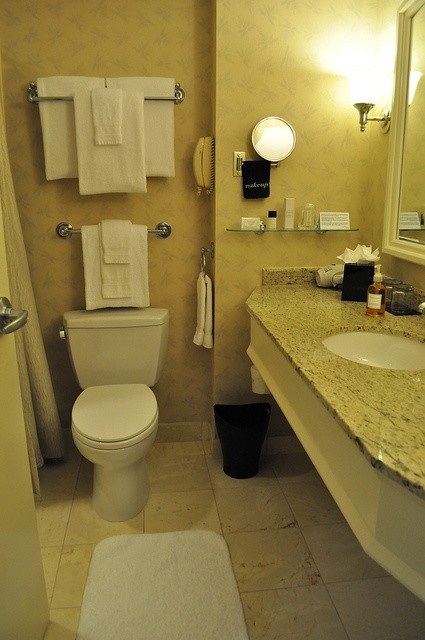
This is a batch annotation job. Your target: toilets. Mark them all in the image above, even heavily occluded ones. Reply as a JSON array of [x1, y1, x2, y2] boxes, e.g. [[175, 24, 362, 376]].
[[63, 308, 169, 522]]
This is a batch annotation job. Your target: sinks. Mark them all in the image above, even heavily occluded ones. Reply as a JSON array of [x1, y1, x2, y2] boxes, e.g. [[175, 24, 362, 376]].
[[321, 324, 425, 371]]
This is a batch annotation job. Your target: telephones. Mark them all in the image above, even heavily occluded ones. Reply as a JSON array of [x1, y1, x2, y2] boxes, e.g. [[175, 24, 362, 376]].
[[193, 136, 215, 198]]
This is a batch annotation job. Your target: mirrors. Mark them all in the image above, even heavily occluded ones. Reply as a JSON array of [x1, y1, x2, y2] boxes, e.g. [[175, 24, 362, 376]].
[[379, 0, 425, 266], [252, 116, 297, 163]]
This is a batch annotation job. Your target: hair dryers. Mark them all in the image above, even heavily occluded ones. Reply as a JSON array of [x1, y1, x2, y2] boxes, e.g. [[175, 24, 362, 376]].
[[316, 263, 344, 286]]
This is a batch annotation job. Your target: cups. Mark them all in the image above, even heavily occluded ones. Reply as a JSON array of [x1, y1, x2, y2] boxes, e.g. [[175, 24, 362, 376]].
[[383, 280, 403, 310], [391, 284, 415, 314]]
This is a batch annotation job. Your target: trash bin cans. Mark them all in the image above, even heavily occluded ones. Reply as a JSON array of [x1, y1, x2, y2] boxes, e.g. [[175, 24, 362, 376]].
[[214, 402, 271, 479]]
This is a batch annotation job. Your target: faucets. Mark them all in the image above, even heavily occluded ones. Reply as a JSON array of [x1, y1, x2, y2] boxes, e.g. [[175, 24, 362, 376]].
[[418, 301, 425, 313]]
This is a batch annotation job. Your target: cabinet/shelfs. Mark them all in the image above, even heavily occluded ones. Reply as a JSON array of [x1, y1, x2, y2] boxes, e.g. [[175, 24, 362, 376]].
[[227, 226, 361, 235]]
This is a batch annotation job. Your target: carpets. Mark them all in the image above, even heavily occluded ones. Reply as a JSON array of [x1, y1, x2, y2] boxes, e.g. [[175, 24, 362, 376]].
[[75, 530, 249, 639]]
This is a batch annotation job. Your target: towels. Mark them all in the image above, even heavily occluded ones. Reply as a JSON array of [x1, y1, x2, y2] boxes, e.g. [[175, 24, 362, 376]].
[[100, 218, 133, 264], [80, 224, 151, 311], [241, 159, 272, 199], [97, 222, 134, 299], [36, 76, 106, 182], [107, 76, 177, 178], [90, 88, 123, 146], [192, 271, 213, 349], [71, 88, 148, 196]]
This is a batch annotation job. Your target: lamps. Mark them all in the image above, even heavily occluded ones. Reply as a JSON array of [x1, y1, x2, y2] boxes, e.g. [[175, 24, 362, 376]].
[[407, 71, 423, 106], [348, 70, 392, 134]]
